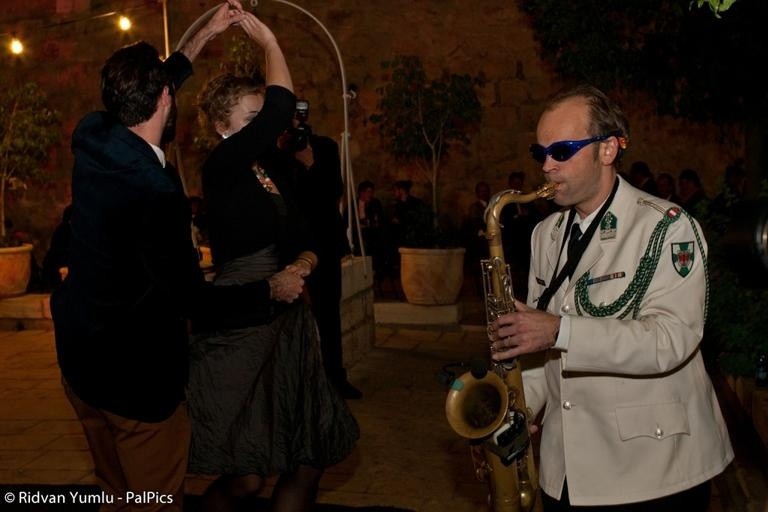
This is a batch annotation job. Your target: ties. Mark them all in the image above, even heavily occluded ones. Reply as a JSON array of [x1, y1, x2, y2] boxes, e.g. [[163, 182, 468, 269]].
[[567, 223, 581, 282]]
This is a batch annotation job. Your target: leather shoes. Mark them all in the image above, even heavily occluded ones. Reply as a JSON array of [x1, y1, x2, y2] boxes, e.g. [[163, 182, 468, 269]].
[[340, 381, 364, 399]]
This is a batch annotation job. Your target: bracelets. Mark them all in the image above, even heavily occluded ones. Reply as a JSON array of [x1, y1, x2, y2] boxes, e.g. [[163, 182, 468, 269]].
[[296, 254, 312, 269]]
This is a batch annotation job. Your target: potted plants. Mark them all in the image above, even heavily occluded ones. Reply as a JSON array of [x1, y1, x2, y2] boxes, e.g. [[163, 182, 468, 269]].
[[1, 78, 64, 298], [370, 51, 486, 308]]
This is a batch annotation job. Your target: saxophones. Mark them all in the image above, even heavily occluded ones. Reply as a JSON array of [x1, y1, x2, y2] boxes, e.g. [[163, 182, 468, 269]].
[[445, 181, 559, 512]]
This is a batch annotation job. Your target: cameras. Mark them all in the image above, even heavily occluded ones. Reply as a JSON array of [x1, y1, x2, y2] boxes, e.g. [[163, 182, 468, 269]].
[[282, 100, 314, 153]]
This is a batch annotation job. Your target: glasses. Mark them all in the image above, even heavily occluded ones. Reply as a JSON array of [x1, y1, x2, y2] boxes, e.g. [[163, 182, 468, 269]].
[[528, 135, 613, 164]]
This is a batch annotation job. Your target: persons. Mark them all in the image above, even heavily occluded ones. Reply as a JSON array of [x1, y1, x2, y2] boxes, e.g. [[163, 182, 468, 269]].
[[45, 0, 244, 512], [193, 11, 359, 512], [352, 173, 539, 237], [473, 84, 736, 511], [269, 108, 362, 398], [620, 158, 768, 262]]
